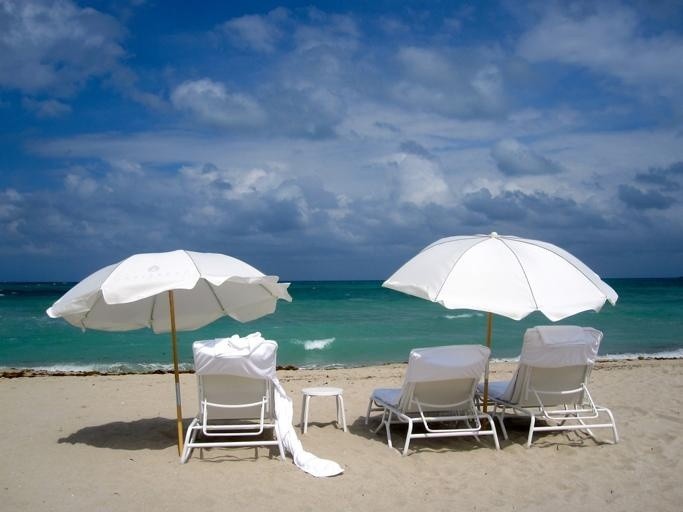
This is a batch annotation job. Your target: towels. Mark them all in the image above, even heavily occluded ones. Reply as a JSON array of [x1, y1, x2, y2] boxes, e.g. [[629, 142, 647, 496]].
[[274, 383, 344, 478], [537, 325, 587, 345], [216, 332, 264, 357]]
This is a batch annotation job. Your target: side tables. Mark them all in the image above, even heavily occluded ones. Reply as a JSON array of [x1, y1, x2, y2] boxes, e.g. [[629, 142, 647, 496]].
[[301, 388, 346, 434]]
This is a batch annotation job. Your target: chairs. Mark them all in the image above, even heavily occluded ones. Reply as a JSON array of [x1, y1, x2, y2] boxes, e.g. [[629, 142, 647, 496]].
[[365, 344, 501, 456], [182, 340, 286, 463], [478, 327, 620, 447]]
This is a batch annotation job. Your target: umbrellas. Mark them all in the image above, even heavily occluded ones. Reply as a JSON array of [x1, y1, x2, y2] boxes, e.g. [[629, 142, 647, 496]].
[[382, 231, 619, 429], [45, 248, 293, 456]]
